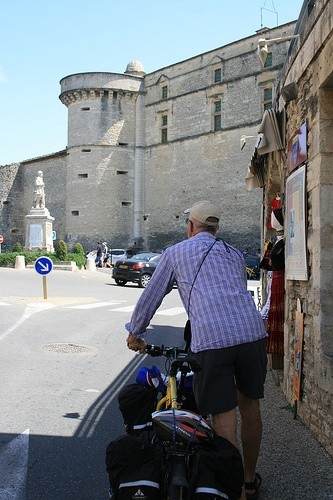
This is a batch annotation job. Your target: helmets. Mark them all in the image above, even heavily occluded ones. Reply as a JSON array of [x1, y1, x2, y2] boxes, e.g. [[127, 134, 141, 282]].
[[151, 406, 214, 448]]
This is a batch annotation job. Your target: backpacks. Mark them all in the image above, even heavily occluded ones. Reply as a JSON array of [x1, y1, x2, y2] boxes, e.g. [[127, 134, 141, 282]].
[[117, 383, 159, 435], [185, 435, 244, 500], [104, 434, 167, 500]]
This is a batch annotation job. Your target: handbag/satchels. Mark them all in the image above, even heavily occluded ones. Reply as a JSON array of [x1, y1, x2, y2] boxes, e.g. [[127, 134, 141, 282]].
[[183, 318, 192, 346]]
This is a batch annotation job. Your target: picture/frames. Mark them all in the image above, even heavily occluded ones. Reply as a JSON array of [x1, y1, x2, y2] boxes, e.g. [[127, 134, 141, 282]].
[[285, 121, 308, 176], [283, 165, 310, 282]]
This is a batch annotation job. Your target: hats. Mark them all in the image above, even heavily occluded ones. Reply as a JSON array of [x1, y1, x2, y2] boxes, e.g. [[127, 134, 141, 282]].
[[102, 241, 107, 247], [184, 200, 224, 228]]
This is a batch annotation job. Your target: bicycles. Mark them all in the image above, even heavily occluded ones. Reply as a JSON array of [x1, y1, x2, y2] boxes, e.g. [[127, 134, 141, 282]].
[[103, 338, 243, 500]]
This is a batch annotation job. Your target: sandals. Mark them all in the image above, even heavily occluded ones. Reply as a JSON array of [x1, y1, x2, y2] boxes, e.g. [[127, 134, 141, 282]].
[[244, 470, 263, 500]]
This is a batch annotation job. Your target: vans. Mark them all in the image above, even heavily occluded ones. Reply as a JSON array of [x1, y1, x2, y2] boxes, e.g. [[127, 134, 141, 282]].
[[101, 250, 133, 267]]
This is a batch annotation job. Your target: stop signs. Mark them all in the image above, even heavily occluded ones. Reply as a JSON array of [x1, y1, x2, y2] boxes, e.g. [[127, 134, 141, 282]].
[[0, 236, 3, 243]]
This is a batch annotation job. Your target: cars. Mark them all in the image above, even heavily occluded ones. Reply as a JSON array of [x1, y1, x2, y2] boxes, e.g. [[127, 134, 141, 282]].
[[113, 253, 178, 289], [240, 255, 261, 279]]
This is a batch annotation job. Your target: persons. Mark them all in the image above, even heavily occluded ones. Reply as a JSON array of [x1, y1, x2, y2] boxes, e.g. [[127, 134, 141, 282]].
[[34, 171, 46, 208], [96, 242, 102, 267], [102, 242, 114, 268], [254, 209, 285, 380], [121, 199, 267, 500]]
